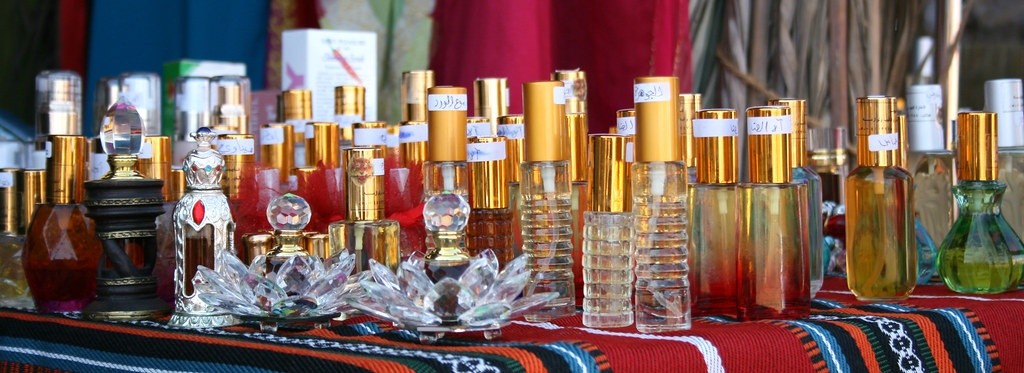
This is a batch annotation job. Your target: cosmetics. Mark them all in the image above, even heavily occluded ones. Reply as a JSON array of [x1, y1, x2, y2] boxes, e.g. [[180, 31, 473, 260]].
[[0, 71, 1023, 333]]
